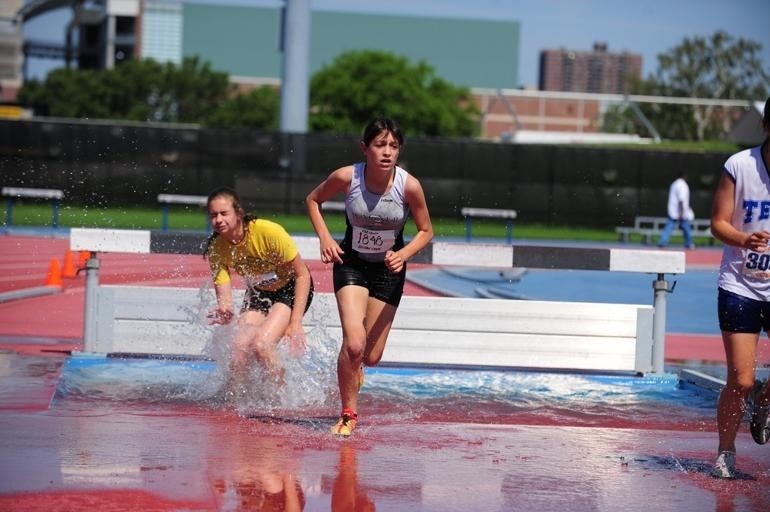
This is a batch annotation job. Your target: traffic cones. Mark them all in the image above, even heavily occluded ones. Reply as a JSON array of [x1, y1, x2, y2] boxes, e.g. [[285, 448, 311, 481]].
[[60, 246, 78, 279], [75, 249, 93, 270], [44, 257, 65, 292]]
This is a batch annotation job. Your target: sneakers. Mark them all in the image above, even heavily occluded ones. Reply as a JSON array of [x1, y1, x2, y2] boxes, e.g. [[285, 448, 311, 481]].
[[750, 383, 770, 445], [358, 366, 364, 388], [331, 408, 358, 436], [711, 451, 736, 480]]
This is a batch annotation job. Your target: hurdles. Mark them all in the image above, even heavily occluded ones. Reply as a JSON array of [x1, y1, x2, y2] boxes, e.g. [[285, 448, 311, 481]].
[[3, 187, 63, 234], [69, 226, 686, 376], [462, 208, 516, 245], [158, 193, 213, 234]]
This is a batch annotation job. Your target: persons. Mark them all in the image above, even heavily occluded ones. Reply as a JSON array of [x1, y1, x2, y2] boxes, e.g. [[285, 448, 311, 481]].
[[206, 421, 305, 512], [656, 168, 697, 251], [330, 438, 376, 511], [199, 187, 315, 401], [708, 95, 769, 478], [304, 116, 433, 435]]
